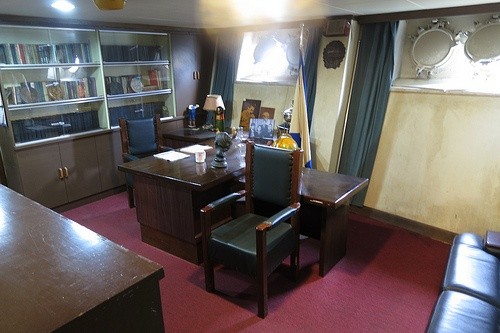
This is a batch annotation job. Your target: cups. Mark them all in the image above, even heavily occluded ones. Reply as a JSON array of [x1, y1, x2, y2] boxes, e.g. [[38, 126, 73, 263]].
[[237, 126, 244, 138], [195, 150, 206, 163], [215, 106, 224, 132]]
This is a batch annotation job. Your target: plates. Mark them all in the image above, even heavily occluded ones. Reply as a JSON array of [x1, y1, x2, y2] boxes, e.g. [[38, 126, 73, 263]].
[[130, 79, 144, 93]]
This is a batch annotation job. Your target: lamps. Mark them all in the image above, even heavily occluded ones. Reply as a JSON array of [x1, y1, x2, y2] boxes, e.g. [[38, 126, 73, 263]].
[[203, 94, 226, 132]]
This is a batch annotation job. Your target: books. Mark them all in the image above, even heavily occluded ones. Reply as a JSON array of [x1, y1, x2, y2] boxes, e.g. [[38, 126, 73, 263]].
[[0, 41, 92, 64], [101, 45, 163, 62], [108, 100, 165, 126], [104, 66, 168, 95], [3, 77, 97, 105], [10, 109, 100, 143]]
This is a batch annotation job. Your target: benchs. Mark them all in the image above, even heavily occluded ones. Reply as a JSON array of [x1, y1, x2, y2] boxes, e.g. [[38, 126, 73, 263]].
[[425, 230, 500, 333]]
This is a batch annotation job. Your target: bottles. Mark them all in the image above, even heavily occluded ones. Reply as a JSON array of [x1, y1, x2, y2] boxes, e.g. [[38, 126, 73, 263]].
[[188, 104, 196, 128]]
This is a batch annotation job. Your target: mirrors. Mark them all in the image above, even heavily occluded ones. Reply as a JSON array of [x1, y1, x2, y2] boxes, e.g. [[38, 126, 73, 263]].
[[408, 19, 462, 79], [464, 15, 500, 80]]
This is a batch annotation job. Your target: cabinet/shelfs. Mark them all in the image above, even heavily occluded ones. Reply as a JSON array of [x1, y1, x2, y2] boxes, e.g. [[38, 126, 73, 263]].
[[171, 32, 214, 116], [0, 25, 110, 149], [98, 30, 176, 130], [15, 136, 101, 209]]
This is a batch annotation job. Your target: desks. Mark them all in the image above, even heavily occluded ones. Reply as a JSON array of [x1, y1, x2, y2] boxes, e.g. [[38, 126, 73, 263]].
[[236, 168, 369, 277], [0, 183, 166, 333], [118, 124, 277, 264]]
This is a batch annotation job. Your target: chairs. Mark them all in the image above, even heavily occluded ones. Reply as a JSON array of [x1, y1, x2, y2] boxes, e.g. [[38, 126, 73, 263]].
[[199, 140, 304, 319], [118, 113, 181, 209]]
